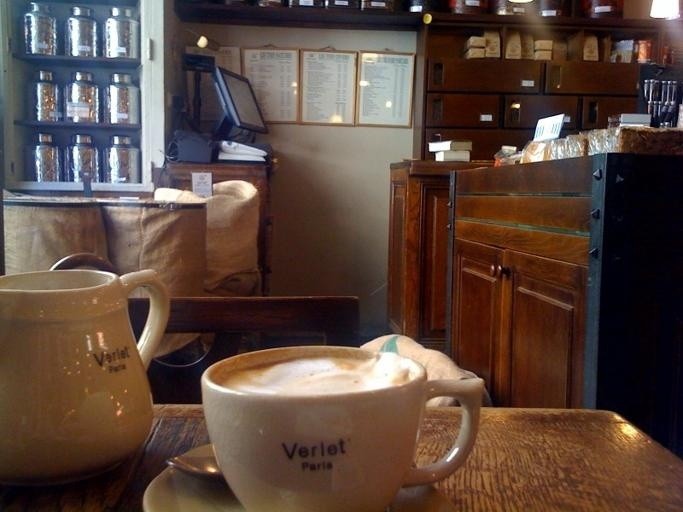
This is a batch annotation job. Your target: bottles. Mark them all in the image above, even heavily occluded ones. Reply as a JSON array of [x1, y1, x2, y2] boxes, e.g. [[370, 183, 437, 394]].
[[101, 6, 140, 60], [63, 7, 102, 57], [100, 134, 140, 182], [20, 1, 61, 56], [102, 71, 141, 125], [24, 70, 63, 123], [63, 134, 100, 182], [63, 71, 101, 123], [21, 132, 63, 182]]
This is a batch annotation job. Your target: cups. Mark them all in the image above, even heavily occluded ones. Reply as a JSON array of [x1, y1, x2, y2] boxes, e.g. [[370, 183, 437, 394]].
[[194, 344, 485, 512], [0, 262, 173, 488]]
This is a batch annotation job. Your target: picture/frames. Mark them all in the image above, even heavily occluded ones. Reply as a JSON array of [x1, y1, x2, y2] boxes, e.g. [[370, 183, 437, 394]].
[[183, 45, 416, 128]]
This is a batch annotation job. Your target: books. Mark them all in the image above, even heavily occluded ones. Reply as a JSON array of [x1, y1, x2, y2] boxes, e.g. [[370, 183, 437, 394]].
[[618, 113, 653, 128], [429, 138, 472, 162]]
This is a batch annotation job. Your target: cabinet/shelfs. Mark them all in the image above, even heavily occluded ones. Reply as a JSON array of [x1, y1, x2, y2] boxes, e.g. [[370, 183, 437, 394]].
[[2, 0, 165, 199], [387, 11, 683, 407], [167, 156, 277, 298]]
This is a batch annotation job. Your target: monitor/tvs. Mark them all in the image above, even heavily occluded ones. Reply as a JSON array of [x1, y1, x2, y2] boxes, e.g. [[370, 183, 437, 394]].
[[213, 65, 269, 135]]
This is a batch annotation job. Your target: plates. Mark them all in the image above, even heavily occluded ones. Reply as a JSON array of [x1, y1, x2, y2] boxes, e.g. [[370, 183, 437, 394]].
[[142, 440, 457, 512]]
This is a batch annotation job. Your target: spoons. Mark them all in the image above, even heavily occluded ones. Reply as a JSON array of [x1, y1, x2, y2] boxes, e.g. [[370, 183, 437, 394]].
[[163, 453, 227, 485]]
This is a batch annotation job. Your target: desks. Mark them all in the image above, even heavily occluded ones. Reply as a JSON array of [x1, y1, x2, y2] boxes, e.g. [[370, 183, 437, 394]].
[[2, 406, 683, 512]]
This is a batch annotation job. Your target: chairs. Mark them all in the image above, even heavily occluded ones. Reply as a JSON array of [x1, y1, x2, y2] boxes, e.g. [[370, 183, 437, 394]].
[[124, 299, 358, 402]]
